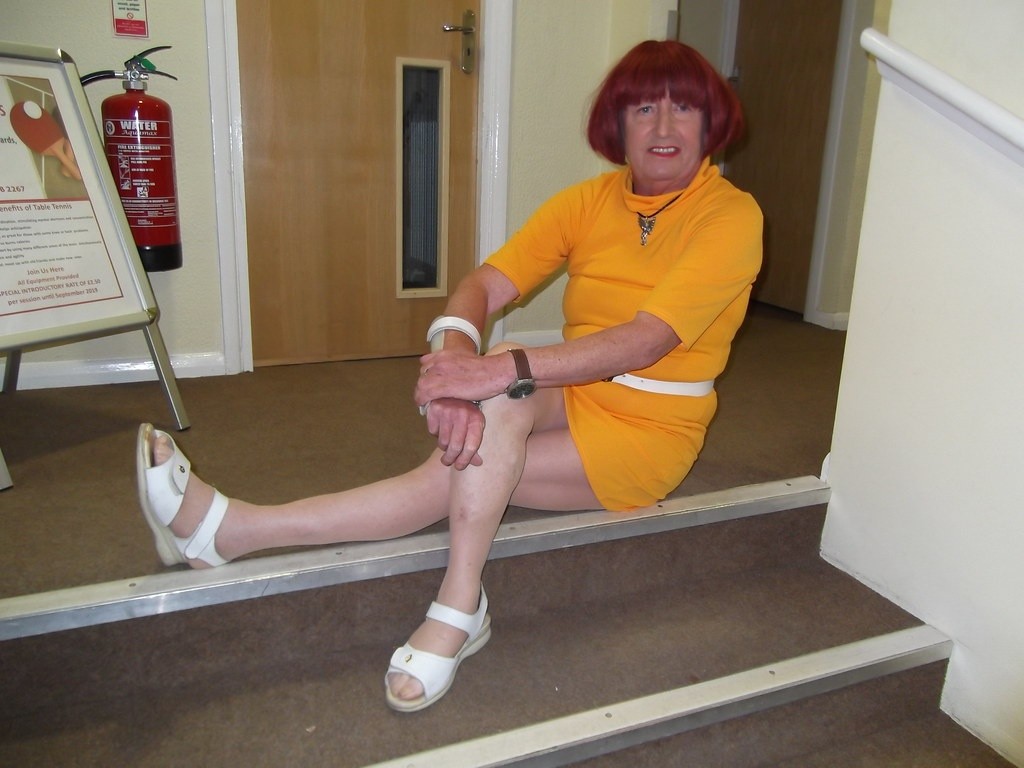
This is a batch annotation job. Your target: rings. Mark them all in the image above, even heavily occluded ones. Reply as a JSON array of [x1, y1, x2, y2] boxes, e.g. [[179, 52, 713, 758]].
[[424, 369, 428, 377]]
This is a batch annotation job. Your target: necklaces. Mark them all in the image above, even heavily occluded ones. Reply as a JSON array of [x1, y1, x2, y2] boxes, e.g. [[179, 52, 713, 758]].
[[635, 192, 684, 247]]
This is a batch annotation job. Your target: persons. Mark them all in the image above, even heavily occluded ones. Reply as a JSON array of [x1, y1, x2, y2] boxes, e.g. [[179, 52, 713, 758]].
[[134, 39, 767, 713]]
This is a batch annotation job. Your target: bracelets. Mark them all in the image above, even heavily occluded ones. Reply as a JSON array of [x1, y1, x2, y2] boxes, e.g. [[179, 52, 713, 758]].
[[426, 316, 482, 354]]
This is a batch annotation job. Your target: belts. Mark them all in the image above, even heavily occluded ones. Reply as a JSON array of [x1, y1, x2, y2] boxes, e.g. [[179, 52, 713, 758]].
[[600, 369, 714, 397]]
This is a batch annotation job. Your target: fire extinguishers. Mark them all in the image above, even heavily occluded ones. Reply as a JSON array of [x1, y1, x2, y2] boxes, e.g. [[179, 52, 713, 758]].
[[81, 44, 191, 272]]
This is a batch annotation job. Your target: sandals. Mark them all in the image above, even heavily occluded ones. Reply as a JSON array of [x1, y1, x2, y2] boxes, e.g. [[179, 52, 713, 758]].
[[384, 580, 491, 712], [135, 422, 232, 567]]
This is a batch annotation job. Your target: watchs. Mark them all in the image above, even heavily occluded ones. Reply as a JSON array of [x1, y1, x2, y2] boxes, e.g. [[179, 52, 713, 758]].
[[506, 349, 537, 400]]
